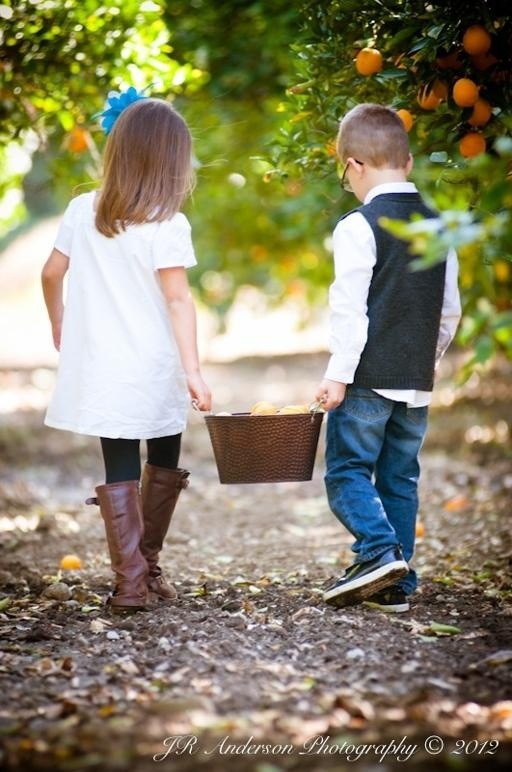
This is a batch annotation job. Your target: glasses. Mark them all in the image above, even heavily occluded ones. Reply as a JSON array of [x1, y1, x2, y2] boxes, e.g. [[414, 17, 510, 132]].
[[337, 156, 364, 195]]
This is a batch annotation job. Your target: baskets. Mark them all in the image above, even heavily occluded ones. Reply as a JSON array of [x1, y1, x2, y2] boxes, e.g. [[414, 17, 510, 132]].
[[200, 408, 328, 486]]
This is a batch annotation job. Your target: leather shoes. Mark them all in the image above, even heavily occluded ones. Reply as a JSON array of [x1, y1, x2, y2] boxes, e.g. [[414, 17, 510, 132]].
[[318, 543, 413, 608], [360, 582, 411, 614]]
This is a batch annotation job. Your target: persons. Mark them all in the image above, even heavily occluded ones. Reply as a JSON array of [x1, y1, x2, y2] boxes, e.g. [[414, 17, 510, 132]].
[[314, 103, 464, 615], [42, 83, 212, 609]]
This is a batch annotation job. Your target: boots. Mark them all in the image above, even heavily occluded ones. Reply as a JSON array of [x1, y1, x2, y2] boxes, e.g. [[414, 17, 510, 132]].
[[141, 460, 192, 602], [94, 478, 161, 616]]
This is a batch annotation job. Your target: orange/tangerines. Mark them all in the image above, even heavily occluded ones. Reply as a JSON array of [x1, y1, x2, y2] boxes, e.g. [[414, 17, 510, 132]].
[[354, 25, 493, 159], [60, 555, 81, 569]]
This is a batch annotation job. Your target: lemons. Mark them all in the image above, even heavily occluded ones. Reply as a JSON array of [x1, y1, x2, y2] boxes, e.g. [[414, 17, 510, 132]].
[[249, 400, 310, 416]]
